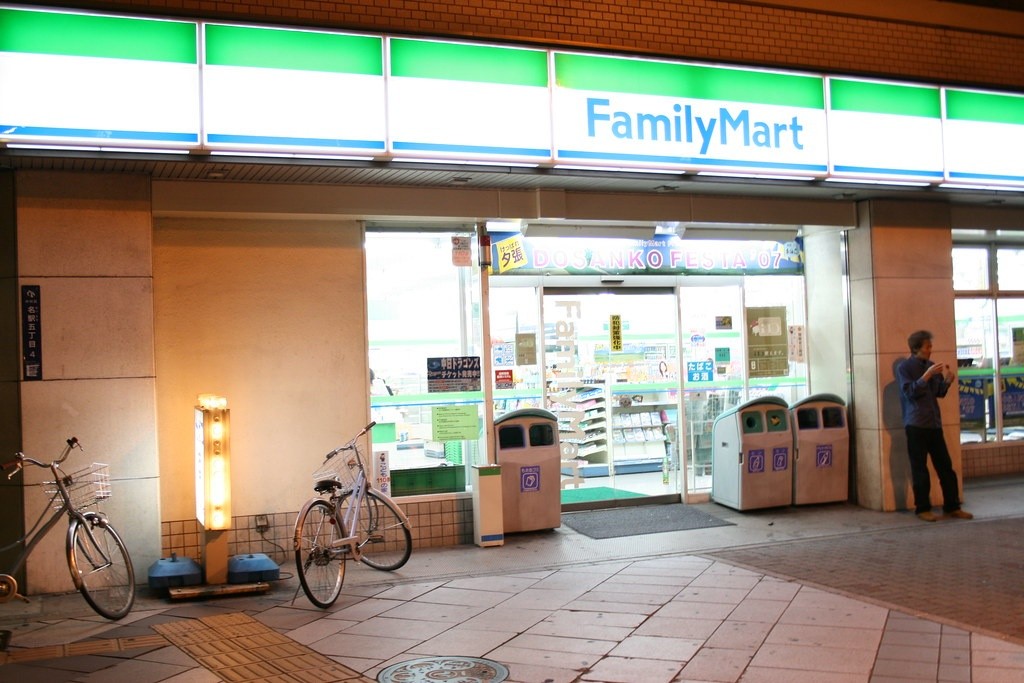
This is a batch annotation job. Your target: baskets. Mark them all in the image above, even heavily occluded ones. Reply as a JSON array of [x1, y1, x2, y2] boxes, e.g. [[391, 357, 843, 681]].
[[312, 443, 368, 496], [40, 463, 111, 513]]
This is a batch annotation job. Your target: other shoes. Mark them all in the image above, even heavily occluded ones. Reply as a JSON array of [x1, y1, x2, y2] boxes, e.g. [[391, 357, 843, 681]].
[[945, 507, 973, 520], [919, 512, 937, 522]]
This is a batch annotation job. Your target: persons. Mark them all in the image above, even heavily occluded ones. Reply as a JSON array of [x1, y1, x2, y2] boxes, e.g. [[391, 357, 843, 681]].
[[896, 331, 973, 522], [659, 362, 667, 379]]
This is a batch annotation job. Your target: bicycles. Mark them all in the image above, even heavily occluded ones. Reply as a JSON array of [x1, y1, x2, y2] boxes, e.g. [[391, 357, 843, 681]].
[[0, 436, 136, 620], [291, 420, 413, 609]]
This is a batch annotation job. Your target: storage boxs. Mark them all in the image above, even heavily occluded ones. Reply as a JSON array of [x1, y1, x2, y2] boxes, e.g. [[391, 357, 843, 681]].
[[471, 463, 506, 549]]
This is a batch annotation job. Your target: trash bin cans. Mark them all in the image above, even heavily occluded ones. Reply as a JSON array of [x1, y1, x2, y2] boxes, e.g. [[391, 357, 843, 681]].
[[478, 407, 563, 535], [788, 392, 849, 506], [709, 394, 793, 512]]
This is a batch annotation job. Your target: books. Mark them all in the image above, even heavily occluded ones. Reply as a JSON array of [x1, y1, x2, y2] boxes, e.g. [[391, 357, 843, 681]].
[[612, 412, 665, 444]]
